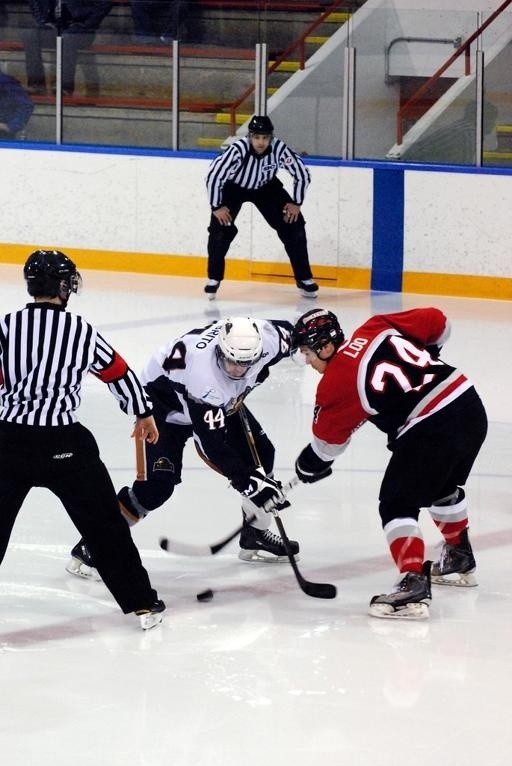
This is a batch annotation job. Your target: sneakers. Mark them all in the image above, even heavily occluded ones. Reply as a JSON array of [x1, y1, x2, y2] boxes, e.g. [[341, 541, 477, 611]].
[[296, 279, 319, 292], [135, 600, 166, 620], [430, 528, 476, 578], [369, 560, 432, 610], [72, 538, 96, 569], [52, 87, 73, 96], [25, 85, 47, 95], [204, 277, 220, 294], [239, 506, 300, 557]]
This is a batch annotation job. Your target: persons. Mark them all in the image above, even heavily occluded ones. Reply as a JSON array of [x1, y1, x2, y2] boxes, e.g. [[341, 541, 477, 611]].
[[66, 315, 301, 584], [294, 306, 490, 621], [0, 69, 34, 140], [24, 0, 96, 97], [0, 248, 167, 630], [202, 114, 321, 301]]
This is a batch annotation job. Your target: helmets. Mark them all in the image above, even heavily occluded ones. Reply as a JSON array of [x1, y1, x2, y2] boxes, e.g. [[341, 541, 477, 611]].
[[24, 250, 75, 280], [291, 308, 338, 348], [218, 318, 263, 368], [248, 115, 274, 133]]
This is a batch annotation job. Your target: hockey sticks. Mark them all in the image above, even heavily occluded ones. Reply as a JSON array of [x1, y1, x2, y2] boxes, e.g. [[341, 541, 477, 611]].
[[160, 475, 301, 559], [237, 403, 338, 600]]
